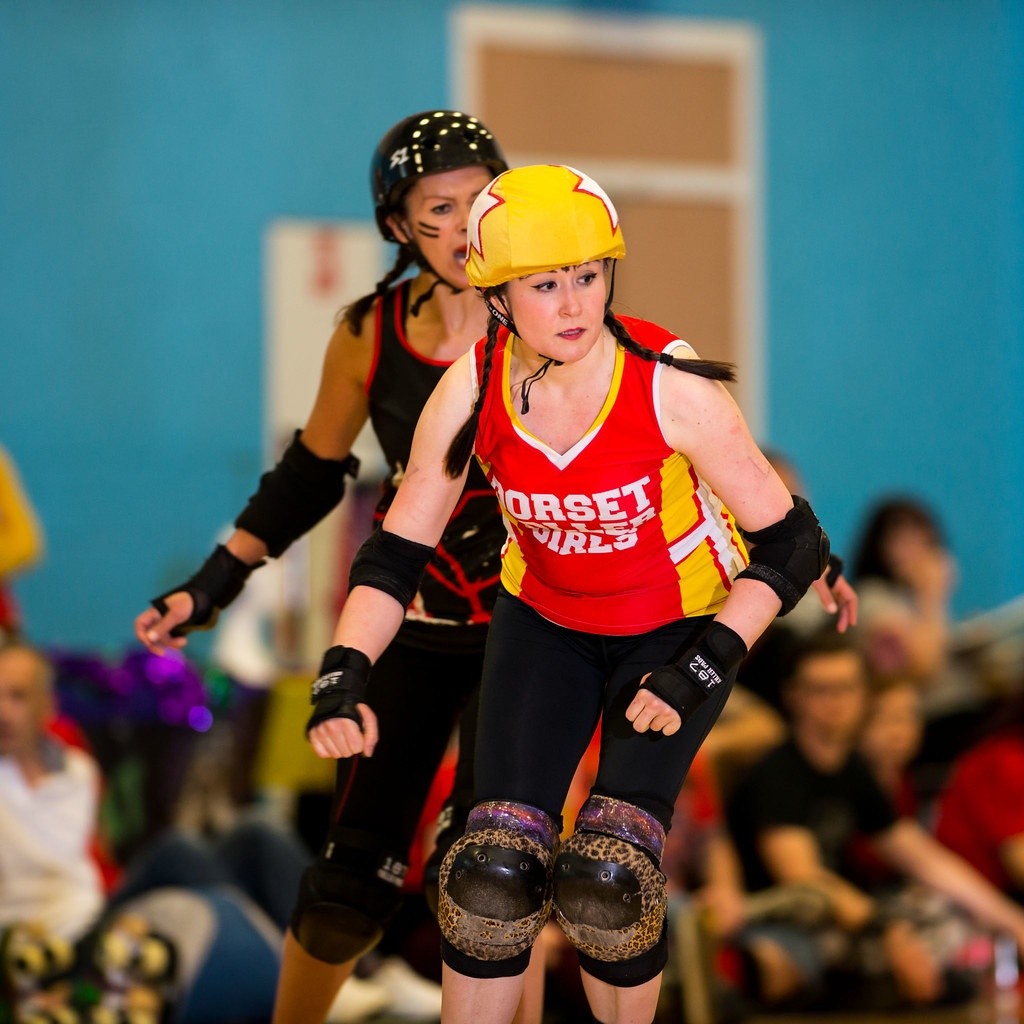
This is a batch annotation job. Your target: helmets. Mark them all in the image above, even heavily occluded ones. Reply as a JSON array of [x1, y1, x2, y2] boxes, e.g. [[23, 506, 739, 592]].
[[369, 109, 507, 242], [465, 164, 626, 288]]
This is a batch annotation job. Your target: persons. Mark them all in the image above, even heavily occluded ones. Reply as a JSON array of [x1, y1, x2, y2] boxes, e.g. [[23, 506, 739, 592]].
[[135, 108, 859, 1023], [302, 162, 831, 1024], [1, 453, 1024, 1024]]
